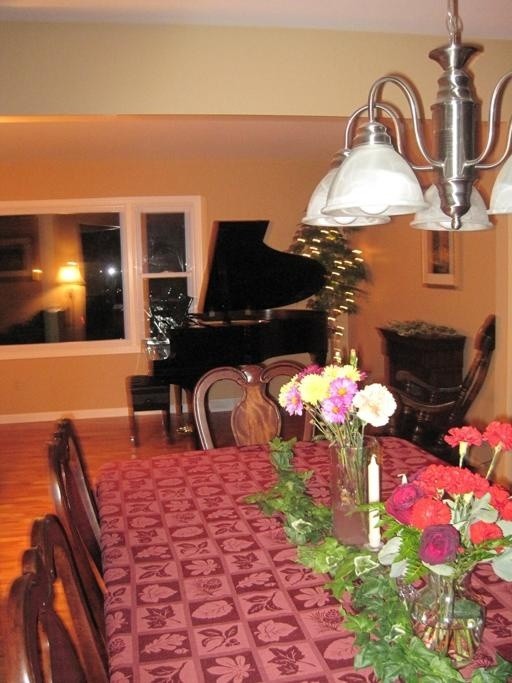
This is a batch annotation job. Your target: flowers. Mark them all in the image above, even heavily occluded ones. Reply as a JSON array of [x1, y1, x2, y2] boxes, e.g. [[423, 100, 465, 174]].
[[344, 421, 512, 663], [279, 349, 397, 535]]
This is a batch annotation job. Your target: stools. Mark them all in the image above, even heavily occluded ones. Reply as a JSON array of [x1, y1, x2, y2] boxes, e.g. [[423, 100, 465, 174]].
[[126, 375, 170, 447]]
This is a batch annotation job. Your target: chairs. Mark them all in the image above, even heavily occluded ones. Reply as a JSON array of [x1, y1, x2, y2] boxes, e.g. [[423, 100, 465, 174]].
[[48, 418, 107, 644], [0, 514, 138, 683], [192, 361, 315, 450], [382, 314, 496, 460]]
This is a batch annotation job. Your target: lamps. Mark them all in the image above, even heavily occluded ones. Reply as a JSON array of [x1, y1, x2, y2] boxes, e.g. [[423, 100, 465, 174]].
[[300, 0, 512, 231], [55, 261, 86, 297]]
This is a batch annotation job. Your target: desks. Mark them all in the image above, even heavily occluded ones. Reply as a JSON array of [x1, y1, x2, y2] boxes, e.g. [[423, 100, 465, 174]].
[[376, 326, 468, 387]]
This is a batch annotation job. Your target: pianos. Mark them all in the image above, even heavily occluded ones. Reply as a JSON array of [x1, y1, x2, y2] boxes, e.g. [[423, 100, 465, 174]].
[[153, 216, 330, 447]]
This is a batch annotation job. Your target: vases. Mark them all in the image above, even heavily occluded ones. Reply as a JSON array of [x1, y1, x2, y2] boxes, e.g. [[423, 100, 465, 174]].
[[411, 573, 485, 669], [327, 435, 376, 546]]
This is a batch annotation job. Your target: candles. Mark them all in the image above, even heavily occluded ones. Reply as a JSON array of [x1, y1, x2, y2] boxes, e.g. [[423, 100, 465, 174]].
[[396, 473, 407, 485], [367, 454, 381, 547]]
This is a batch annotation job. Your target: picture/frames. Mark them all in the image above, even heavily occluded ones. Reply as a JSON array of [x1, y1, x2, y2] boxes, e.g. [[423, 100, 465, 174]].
[[0, 236, 36, 282], [421, 230, 461, 289]]
